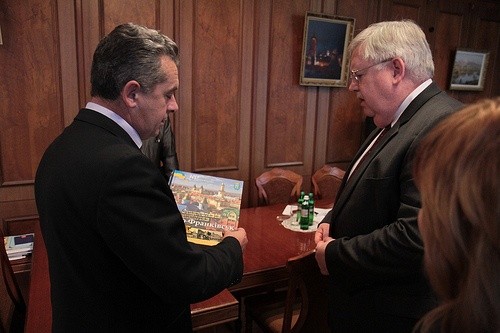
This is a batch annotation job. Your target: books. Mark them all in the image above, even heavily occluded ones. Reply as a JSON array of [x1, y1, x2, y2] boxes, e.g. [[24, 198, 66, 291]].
[[4, 234, 34, 261], [167, 169, 244, 246]]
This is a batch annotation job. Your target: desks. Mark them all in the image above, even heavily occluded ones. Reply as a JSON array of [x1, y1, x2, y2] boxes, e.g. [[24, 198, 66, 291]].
[[25, 193, 340, 333]]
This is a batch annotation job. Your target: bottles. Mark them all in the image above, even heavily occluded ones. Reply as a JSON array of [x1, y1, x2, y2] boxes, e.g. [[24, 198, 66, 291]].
[[296, 191, 315, 230]]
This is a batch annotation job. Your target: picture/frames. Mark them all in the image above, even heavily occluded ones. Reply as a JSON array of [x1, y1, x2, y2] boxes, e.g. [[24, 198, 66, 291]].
[[447, 48, 491, 92], [298, 10, 357, 88]]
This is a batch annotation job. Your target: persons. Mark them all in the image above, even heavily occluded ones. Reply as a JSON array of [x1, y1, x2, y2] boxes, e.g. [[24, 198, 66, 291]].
[[313, 19, 469, 333], [415, 96, 500, 333], [34, 22, 248, 333]]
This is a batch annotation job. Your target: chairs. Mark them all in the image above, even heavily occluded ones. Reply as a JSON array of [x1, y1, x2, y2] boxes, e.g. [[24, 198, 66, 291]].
[[0, 217, 28, 333], [243, 248, 331, 333], [310, 164, 347, 198], [254, 168, 304, 207]]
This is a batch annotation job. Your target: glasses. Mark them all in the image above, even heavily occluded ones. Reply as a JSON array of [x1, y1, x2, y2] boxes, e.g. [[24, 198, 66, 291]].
[[349, 57, 394, 82]]
